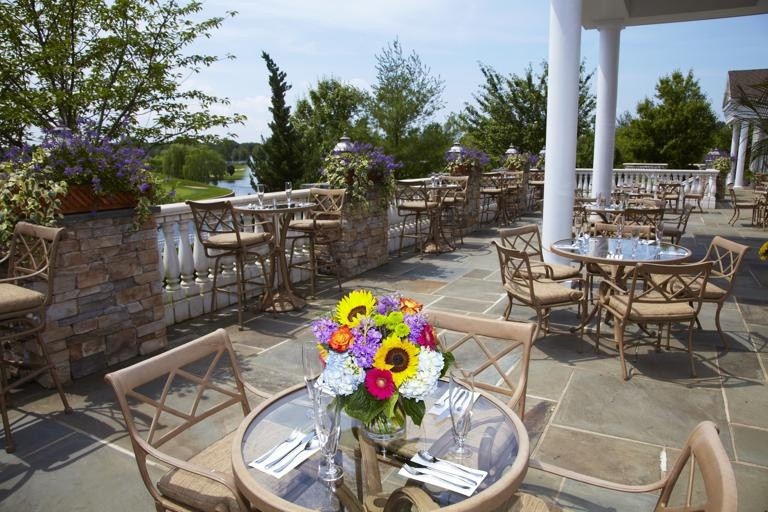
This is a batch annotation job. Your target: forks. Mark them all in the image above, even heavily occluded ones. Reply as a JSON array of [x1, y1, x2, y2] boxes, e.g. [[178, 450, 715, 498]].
[[256, 426, 301, 463], [402, 463, 470, 491]]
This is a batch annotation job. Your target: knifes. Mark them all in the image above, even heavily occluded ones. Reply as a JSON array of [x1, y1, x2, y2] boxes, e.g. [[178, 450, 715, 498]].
[[404, 458, 477, 486], [432, 391, 466, 424], [266, 431, 316, 470]]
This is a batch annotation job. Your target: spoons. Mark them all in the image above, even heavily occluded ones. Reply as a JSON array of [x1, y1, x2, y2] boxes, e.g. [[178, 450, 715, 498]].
[[419, 450, 484, 479], [274, 434, 322, 472], [434, 393, 451, 408]]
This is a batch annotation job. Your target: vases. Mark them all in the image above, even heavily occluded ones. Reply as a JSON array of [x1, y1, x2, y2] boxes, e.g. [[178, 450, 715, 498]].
[[448, 163, 479, 176], [7, 182, 154, 216], [327, 167, 379, 186], [352, 382, 411, 445]]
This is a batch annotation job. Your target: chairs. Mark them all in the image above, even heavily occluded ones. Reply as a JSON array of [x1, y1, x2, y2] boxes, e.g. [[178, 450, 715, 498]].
[[484, 421, 737, 512], [185, 200, 278, 330], [0, 222, 72, 453], [432, 175, 469, 251], [490, 241, 585, 348], [598, 263, 713, 382], [659, 204, 695, 245], [416, 311, 536, 411], [393, 179, 436, 258], [498, 224, 582, 321], [658, 183, 681, 213], [480, 173, 510, 227], [647, 234, 752, 350], [586, 222, 652, 318], [287, 187, 348, 301], [683, 182, 709, 213], [104, 327, 256, 512], [728, 189, 759, 227]]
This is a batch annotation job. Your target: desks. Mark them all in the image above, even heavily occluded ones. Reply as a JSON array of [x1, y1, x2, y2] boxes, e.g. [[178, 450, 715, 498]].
[[231, 372, 529, 512], [551, 235, 690, 335], [231, 200, 315, 310], [411, 183, 457, 253], [488, 176, 519, 224]]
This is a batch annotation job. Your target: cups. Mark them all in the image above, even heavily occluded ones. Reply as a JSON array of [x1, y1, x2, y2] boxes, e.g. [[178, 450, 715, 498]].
[[632, 229, 640, 258], [302, 341, 324, 400], [618, 215, 625, 241], [448, 369, 474, 459], [575, 216, 582, 241], [583, 222, 592, 251], [655, 221, 664, 248], [285, 182, 292, 208], [257, 183, 264, 208], [312, 385, 345, 483]]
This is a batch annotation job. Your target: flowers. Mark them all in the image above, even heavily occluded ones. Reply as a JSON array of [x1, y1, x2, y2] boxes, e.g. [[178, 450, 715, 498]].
[[1, 119, 159, 236], [310, 288, 455, 434], [317, 141, 400, 208], [444, 147, 490, 176]]
